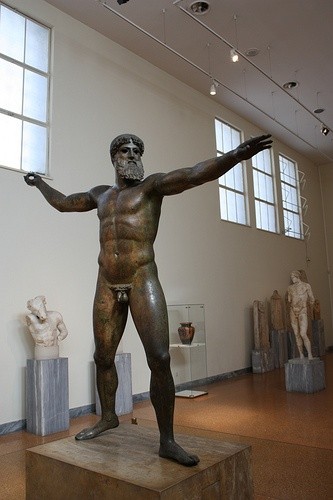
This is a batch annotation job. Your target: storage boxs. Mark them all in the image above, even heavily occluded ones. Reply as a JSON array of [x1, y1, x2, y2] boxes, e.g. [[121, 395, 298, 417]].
[[167, 303, 209, 399]]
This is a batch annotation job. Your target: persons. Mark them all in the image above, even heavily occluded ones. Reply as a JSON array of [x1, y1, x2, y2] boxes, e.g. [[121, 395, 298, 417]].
[[25, 296, 68, 359], [22, 134, 273, 466], [287, 271, 315, 360], [270, 289, 286, 368]]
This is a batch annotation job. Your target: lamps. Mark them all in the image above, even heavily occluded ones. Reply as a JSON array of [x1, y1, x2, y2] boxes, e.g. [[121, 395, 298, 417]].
[[319, 124, 330, 136], [229, 48, 240, 63], [208, 85, 217, 95]]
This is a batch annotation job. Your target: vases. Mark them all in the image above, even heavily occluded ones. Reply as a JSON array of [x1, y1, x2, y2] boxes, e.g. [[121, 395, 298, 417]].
[[177, 323, 195, 344]]
